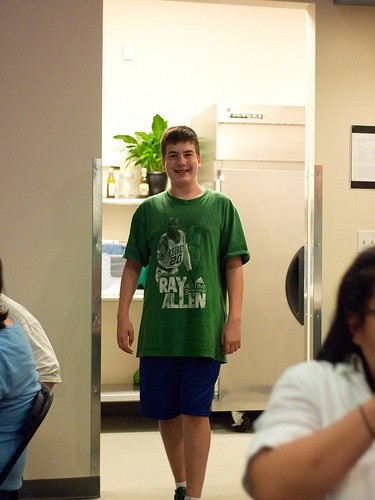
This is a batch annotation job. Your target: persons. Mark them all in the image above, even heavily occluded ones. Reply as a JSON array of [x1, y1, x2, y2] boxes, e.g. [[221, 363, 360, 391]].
[[240, 247, 375, 500], [0, 259, 62, 500], [116, 126, 250, 500]]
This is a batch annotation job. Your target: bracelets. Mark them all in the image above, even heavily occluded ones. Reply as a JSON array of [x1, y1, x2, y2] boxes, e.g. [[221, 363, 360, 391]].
[[358, 404, 375, 438]]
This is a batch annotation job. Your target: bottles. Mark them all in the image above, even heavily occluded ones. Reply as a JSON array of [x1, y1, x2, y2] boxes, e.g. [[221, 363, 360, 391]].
[[105, 169, 116, 199]]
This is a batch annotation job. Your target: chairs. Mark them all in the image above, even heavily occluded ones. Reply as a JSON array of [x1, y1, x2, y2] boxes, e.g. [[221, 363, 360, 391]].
[[0, 381, 54, 487]]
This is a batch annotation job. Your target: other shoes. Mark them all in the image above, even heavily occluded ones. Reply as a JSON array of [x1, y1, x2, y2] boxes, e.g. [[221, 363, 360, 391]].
[[174, 487, 185, 500]]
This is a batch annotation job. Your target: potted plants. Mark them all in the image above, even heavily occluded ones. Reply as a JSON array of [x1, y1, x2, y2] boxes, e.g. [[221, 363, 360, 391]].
[[113, 114, 170, 194]]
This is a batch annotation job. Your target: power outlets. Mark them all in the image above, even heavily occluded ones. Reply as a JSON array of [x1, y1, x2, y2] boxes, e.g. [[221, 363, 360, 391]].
[[358, 230, 375, 253]]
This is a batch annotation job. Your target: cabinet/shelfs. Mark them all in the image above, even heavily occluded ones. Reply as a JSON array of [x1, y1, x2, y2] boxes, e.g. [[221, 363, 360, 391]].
[[102, 196, 145, 402]]
